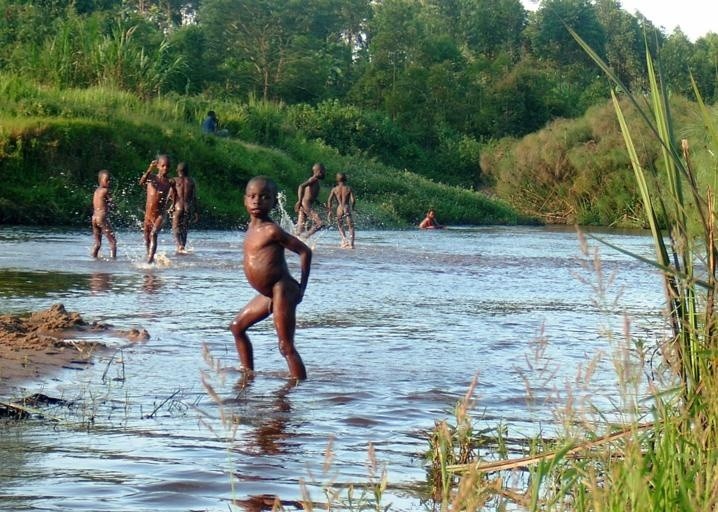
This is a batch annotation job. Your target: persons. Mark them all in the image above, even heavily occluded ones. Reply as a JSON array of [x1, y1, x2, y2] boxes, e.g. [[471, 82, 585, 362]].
[[229, 176, 312, 380], [327, 172, 356, 249], [294, 163, 326, 239], [140, 155, 170, 264], [203, 111, 229, 137], [170, 162, 199, 252], [90, 170, 117, 258], [419, 210, 444, 229]]
